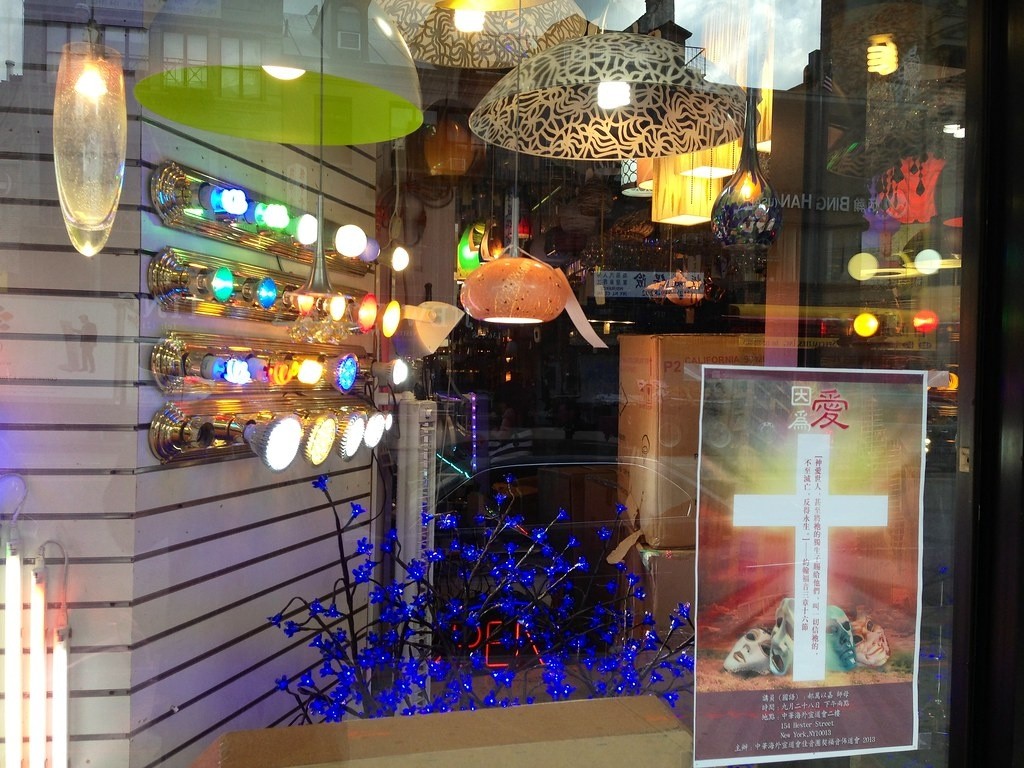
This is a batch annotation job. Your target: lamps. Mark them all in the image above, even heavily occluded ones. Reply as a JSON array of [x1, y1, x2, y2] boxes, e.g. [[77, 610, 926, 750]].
[[52, 0, 128, 258], [133, 0, 823, 473], [0, 473, 73, 768]]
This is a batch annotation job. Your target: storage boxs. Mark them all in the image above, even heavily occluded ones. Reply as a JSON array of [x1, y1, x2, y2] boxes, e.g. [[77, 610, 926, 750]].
[[540, 334, 766, 651]]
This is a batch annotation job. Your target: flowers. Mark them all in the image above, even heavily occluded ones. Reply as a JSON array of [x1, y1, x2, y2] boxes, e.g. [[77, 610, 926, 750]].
[[273, 471, 701, 725]]
